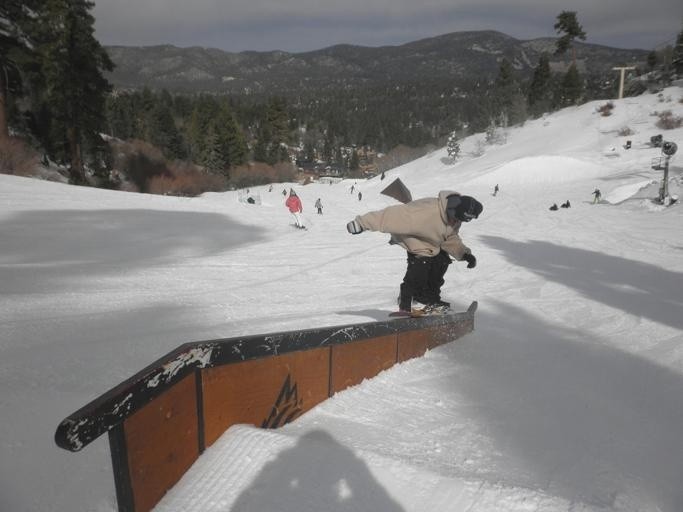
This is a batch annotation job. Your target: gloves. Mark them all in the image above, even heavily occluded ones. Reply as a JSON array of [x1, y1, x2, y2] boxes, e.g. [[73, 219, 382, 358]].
[[465, 255, 477, 269], [347, 220, 363, 235]]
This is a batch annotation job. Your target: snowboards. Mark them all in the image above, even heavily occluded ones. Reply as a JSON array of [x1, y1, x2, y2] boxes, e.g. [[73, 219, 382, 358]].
[[388, 298, 450, 319], [291, 222, 308, 231]]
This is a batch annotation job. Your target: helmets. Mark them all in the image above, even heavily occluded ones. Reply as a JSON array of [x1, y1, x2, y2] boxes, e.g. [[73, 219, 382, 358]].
[[455, 194, 483, 222]]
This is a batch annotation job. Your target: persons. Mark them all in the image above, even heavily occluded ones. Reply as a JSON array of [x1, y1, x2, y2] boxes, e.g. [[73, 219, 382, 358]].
[[346, 189, 483, 316], [590, 187, 601, 204], [285, 189, 303, 228], [492, 183, 498, 196], [281, 189, 287, 195], [549, 204, 558, 210], [357, 192, 362, 200], [289, 188, 296, 195], [348, 185, 355, 193], [380, 171, 384, 180], [560, 199, 570, 207], [314, 197, 323, 213], [247, 197, 254, 205], [246, 189, 248, 194], [268, 184, 272, 193]]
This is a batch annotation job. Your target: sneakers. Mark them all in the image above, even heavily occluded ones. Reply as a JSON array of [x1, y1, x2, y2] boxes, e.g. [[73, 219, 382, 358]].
[[430, 300, 450, 312], [410, 302, 443, 317]]
